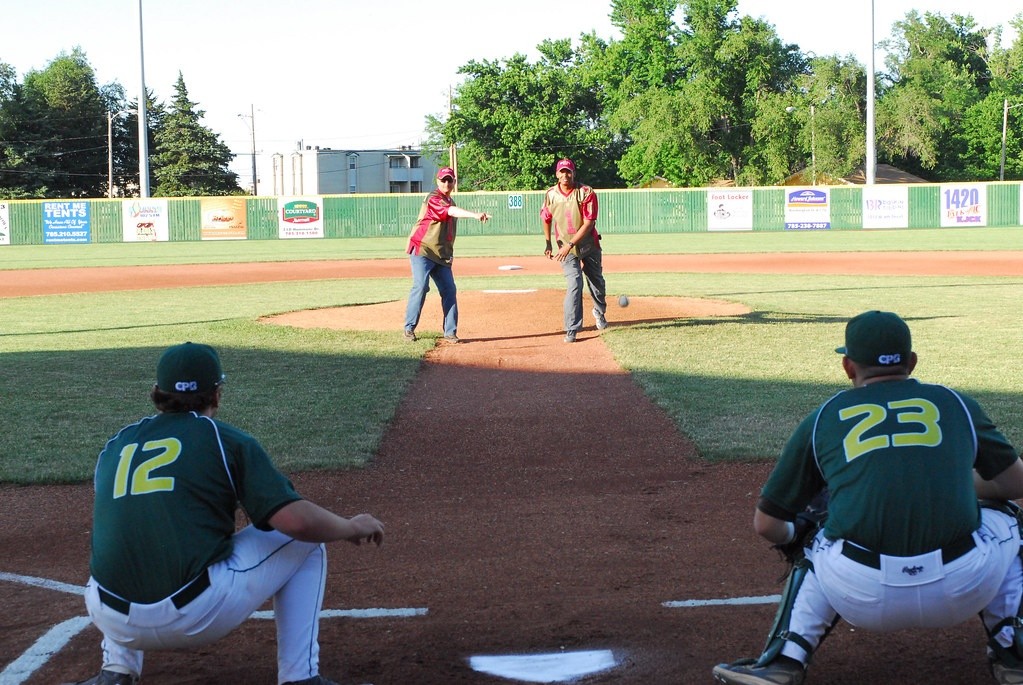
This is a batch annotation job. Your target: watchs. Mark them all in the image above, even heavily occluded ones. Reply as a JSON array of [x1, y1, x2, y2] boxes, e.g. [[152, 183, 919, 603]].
[[567, 241, 574, 248]]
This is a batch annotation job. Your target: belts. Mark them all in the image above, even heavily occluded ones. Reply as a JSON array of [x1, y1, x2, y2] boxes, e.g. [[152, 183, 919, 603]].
[[841, 535, 977, 570], [97, 569, 211, 617]]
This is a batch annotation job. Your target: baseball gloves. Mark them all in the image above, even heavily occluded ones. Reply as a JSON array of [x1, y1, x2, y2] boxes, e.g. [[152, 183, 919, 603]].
[[767, 487, 832, 584]]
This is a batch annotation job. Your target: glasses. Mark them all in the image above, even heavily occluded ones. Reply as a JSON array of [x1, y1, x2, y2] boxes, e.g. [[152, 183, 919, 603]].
[[440, 177, 453, 183]]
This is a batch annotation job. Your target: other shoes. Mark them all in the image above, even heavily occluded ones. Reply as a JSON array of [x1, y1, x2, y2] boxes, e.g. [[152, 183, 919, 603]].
[[403, 330, 417, 341], [593, 307, 608, 330], [987, 626, 1023, 685], [62, 668, 134, 685], [444, 335, 460, 344], [282, 674, 339, 685], [712, 653, 808, 685], [564, 329, 576, 343]]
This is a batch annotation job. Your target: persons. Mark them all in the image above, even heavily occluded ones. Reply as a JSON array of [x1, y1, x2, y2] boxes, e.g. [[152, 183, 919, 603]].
[[84, 342, 384, 685], [540, 158, 608, 343], [713, 310, 1023, 685], [402, 168, 493, 344]]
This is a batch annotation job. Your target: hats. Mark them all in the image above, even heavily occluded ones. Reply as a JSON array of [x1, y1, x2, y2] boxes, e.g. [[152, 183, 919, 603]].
[[835, 310, 912, 365], [437, 167, 456, 181], [155, 340, 225, 396], [556, 159, 574, 173]]
